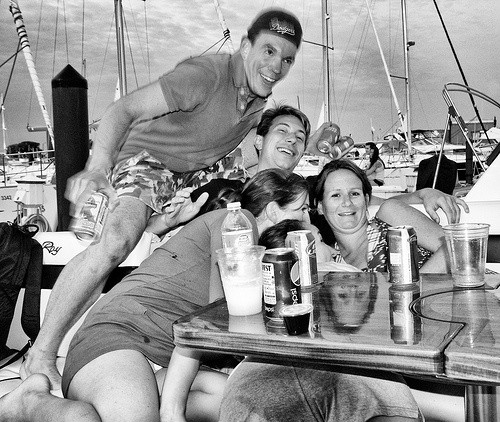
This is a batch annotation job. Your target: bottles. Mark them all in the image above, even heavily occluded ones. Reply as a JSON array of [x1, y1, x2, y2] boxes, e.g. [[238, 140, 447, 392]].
[[221, 202, 260, 249]]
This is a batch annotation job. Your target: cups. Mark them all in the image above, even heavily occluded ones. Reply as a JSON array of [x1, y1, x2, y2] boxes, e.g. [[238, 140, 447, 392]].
[[216, 246, 266, 316], [279, 304, 313, 336], [441, 223, 489, 288]]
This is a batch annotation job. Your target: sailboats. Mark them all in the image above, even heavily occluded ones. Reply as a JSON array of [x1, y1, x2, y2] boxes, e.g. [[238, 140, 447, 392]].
[[0, 1, 500, 360]]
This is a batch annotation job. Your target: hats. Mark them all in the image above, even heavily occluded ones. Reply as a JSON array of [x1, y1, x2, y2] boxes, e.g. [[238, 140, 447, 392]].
[[247, 7, 304, 50]]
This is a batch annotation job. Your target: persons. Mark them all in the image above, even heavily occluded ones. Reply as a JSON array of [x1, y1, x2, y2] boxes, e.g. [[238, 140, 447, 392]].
[[1, 8, 500, 421], [318, 271, 379, 336]]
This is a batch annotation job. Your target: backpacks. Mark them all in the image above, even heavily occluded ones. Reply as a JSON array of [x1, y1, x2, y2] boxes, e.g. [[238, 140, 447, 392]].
[[1, 216, 43, 372]]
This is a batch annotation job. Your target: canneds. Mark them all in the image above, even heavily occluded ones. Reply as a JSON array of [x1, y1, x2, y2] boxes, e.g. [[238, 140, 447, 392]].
[[261, 247, 302, 321], [386, 225, 421, 285], [284, 229, 319, 288], [73, 192, 108, 242], [301, 286, 321, 339], [387, 283, 422, 345], [317, 123, 354, 159]]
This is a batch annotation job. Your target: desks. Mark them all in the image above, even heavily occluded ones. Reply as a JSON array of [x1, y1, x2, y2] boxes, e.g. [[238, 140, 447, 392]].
[[171, 272, 500, 422]]
